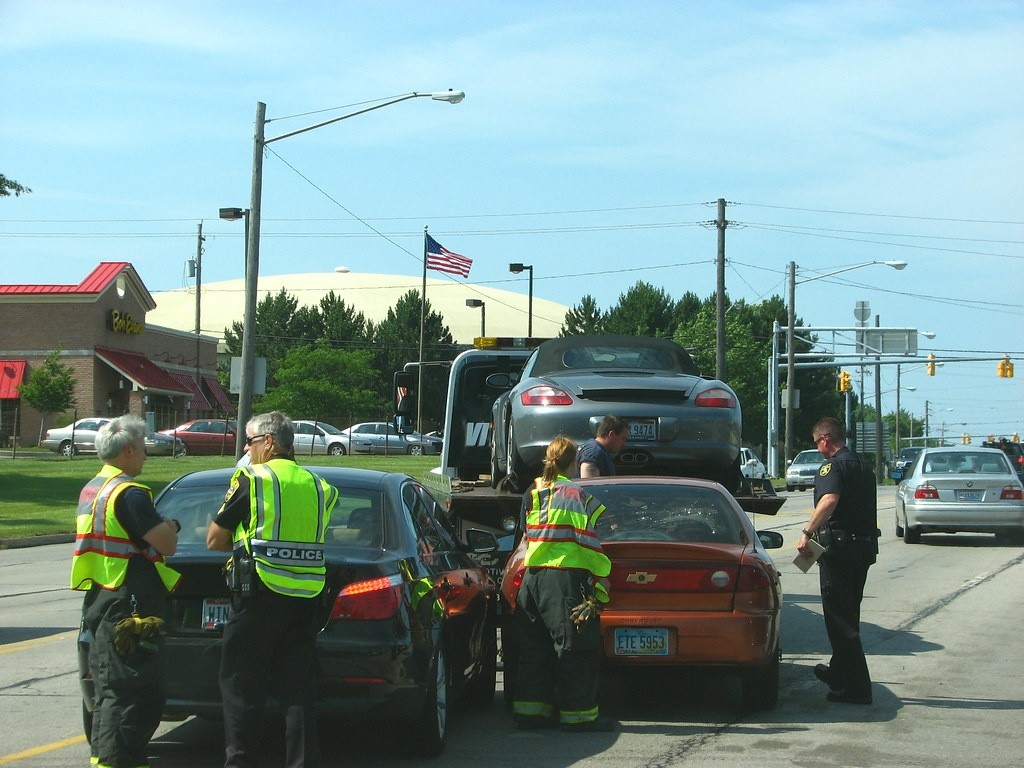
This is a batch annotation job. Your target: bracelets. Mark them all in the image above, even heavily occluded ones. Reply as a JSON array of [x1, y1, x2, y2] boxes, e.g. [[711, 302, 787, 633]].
[[172, 519, 181, 534]]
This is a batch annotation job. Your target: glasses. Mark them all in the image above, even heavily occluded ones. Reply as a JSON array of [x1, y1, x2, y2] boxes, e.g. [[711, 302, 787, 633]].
[[813, 432, 831, 449], [245, 433, 278, 446]]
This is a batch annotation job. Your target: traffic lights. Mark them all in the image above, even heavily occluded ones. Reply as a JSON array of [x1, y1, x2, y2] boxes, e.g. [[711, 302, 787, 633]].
[[840, 371, 852, 392]]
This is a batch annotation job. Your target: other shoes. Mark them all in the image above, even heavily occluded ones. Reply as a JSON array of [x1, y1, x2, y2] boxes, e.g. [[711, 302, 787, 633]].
[[560, 713, 620, 732], [827, 688, 872, 705], [517, 716, 560, 731], [814, 664, 838, 691]]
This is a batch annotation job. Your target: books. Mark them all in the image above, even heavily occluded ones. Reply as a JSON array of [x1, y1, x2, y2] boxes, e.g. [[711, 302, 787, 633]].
[[792, 539, 825, 574]]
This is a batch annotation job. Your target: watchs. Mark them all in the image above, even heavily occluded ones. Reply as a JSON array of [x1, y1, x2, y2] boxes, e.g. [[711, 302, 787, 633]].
[[802, 528, 813, 538]]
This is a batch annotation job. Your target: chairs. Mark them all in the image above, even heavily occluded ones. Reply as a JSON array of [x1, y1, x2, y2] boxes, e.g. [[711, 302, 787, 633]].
[[930, 463, 948, 472], [347, 506, 376, 542], [982, 464, 999, 472]]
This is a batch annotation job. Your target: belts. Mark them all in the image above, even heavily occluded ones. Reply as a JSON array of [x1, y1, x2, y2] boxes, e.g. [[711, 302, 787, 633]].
[[818, 525, 882, 536]]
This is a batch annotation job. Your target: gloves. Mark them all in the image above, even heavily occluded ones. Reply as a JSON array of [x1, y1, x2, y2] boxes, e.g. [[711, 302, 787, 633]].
[[569, 596, 604, 635], [113, 615, 164, 656]]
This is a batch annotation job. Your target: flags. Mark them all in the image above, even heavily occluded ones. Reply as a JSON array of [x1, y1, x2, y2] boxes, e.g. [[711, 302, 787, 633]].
[[425, 233, 473, 279]]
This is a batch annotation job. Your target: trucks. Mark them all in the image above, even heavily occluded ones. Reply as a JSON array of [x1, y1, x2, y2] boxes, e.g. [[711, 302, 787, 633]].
[[393, 337, 788, 552]]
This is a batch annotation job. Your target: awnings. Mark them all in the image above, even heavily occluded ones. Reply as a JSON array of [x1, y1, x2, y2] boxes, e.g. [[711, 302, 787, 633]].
[[170, 373, 213, 411], [202, 377, 235, 412], [94, 347, 195, 399], [0, 360, 26, 398]]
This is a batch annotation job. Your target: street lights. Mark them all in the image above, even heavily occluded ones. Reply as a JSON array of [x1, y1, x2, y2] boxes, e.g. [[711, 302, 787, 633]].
[[509, 262, 533, 337], [896, 363, 968, 460], [860, 387, 917, 454], [466, 299, 485, 337], [218, 207, 250, 280], [234, 89, 465, 468], [874, 331, 937, 481], [786, 260, 908, 480]]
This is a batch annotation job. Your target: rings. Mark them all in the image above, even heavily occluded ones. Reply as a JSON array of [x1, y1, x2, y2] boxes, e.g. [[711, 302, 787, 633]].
[[803, 551, 805, 554]]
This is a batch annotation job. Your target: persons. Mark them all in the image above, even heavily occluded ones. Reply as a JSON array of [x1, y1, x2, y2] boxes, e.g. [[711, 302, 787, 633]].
[[576, 415, 630, 537], [797, 418, 882, 706], [206, 411, 338, 768], [509, 438, 615, 732], [70, 417, 181, 768]]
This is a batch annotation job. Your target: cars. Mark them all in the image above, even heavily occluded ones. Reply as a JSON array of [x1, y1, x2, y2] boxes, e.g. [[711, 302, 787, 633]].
[[41, 418, 187, 457], [497, 474, 785, 712], [894, 444, 1022, 544], [785, 449, 828, 493], [341, 421, 444, 456], [157, 419, 246, 456], [740, 448, 766, 479], [291, 420, 377, 456], [979, 439, 1024, 480], [895, 447, 929, 472], [82, 465, 497, 758]]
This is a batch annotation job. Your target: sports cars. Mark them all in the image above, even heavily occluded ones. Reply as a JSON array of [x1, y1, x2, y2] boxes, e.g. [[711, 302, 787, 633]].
[[486, 334, 743, 494]]
